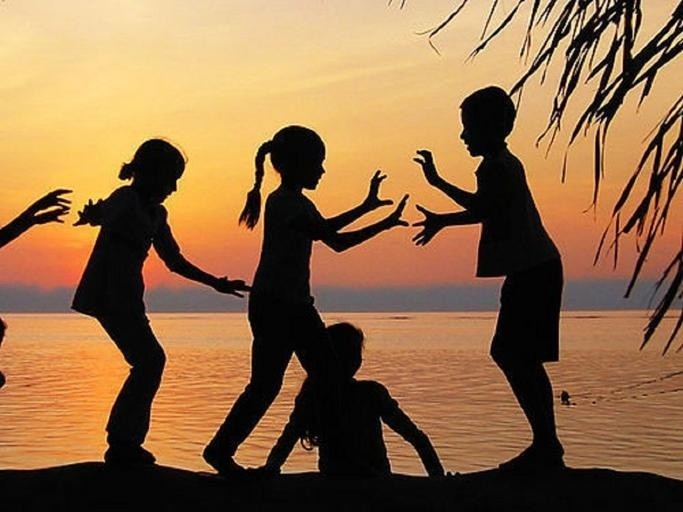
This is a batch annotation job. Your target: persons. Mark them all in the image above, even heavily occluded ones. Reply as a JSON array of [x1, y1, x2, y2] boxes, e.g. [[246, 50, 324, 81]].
[[411, 86, 566, 474], [0, 187, 74, 388], [245, 320, 445, 485], [70, 138, 253, 463], [201, 124, 411, 479]]
